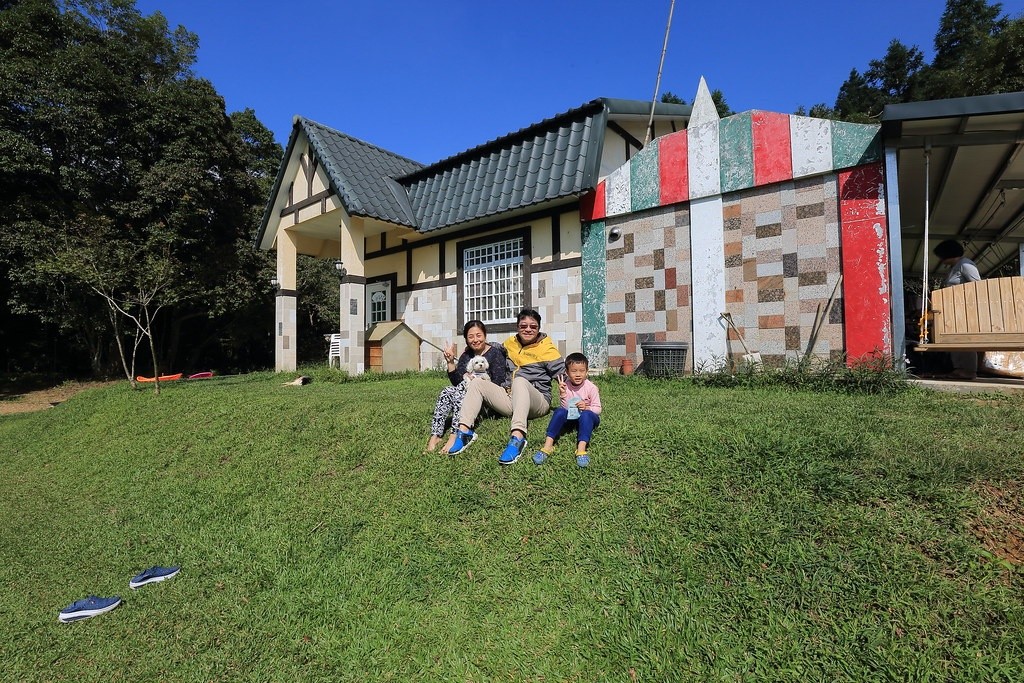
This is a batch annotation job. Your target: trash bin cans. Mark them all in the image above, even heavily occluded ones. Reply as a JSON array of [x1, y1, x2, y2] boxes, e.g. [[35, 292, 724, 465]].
[[641, 341, 689, 378]]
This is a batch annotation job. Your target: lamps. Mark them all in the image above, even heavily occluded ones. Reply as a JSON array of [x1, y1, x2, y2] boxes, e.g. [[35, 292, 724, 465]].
[[334, 257, 347, 281], [270, 276, 281, 291]]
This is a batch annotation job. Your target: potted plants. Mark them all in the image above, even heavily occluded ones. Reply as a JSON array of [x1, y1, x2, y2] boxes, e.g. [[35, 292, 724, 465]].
[[904, 272, 945, 313]]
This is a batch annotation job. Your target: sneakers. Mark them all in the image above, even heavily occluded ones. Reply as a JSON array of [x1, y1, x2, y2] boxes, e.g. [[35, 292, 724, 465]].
[[129, 565, 180, 590], [499, 435, 528, 464], [59, 595, 122, 624], [448, 428, 478, 455]]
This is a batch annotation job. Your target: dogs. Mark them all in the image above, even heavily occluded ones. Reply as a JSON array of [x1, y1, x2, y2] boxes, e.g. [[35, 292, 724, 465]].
[[462, 354, 490, 384]]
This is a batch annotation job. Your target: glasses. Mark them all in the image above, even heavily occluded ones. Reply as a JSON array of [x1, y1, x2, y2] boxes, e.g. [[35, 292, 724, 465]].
[[517, 324, 539, 329]]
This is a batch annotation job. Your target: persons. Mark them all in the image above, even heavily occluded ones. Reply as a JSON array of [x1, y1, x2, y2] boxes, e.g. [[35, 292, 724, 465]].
[[448, 309, 568, 465], [531, 352, 601, 467], [931, 240, 982, 380], [425, 319, 506, 454]]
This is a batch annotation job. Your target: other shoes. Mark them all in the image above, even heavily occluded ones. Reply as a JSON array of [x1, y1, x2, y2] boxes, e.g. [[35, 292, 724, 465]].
[[941, 370, 976, 382]]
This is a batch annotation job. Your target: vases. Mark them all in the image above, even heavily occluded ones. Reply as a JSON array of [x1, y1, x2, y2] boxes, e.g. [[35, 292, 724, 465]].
[[623, 358, 635, 375]]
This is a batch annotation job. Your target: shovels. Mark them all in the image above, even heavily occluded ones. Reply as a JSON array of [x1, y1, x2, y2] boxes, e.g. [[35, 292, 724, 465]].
[[721, 312, 764, 372]]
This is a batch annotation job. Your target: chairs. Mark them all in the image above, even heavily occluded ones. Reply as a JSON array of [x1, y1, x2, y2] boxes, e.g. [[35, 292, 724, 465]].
[[328, 334, 341, 368]]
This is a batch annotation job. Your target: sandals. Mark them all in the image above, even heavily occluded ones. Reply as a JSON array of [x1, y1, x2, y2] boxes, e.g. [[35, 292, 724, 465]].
[[532, 446, 555, 465], [575, 449, 590, 467]]
